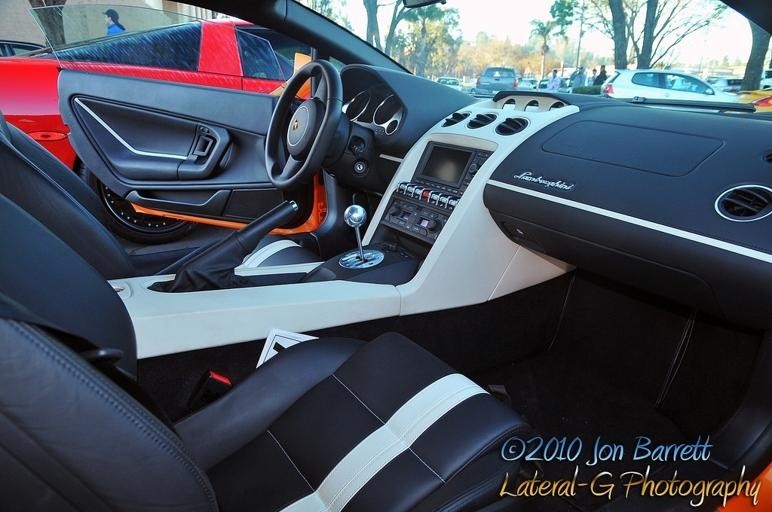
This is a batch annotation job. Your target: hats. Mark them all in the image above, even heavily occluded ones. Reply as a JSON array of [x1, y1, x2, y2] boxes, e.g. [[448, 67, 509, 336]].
[[102, 9, 119, 19]]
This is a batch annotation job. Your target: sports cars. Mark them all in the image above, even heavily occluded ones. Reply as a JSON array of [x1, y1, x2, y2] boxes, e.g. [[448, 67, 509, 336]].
[[0, 17, 319, 245]]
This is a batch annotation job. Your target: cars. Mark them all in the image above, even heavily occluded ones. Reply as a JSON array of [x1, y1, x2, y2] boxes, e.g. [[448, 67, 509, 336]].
[[0, 40, 46, 61], [432, 61, 772, 115]]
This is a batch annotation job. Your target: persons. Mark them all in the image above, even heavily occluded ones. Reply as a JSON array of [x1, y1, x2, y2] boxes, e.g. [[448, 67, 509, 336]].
[[545, 69, 561, 90], [101, 9, 126, 35], [593, 65, 607, 86], [587, 69, 598, 87], [570, 66, 586, 89]]
